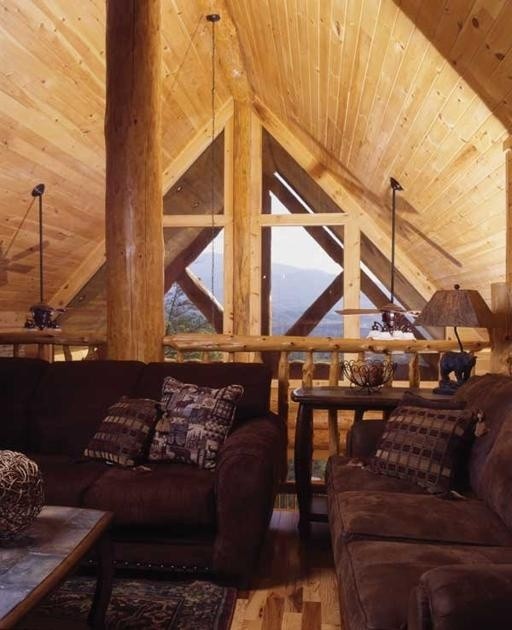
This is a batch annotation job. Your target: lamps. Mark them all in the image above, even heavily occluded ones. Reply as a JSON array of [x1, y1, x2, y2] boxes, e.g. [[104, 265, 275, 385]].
[[371, 177, 414, 334], [409, 283, 499, 351], [24, 185, 66, 330]]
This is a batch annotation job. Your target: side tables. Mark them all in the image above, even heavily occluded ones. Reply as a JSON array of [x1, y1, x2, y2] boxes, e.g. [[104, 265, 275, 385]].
[[290, 386, 454, 540]]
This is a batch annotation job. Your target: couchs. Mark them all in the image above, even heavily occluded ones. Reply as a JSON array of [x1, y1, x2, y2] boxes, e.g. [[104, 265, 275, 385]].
[[0, 412, 289, 592], [324, 417, 512, 629]]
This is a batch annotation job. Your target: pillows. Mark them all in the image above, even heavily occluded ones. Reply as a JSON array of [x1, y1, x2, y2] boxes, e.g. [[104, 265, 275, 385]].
[[369, 372, 512, 537], [0, 356, 274, 475]]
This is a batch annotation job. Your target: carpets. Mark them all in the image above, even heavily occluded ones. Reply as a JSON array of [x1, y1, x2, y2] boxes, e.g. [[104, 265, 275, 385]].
[[0, 565, 237, 630]]
